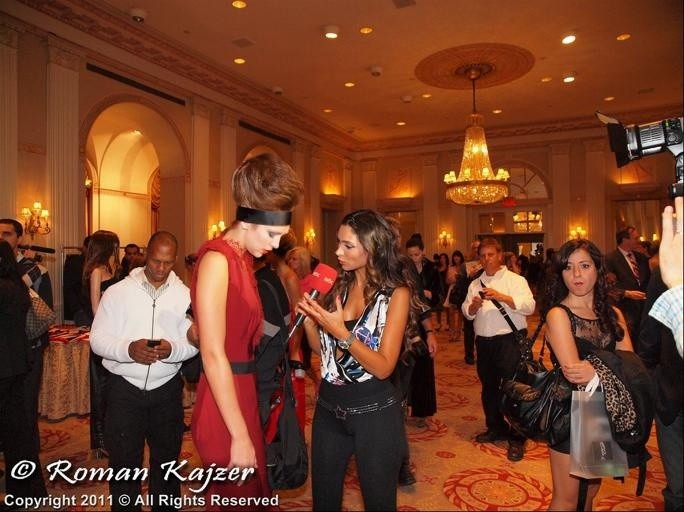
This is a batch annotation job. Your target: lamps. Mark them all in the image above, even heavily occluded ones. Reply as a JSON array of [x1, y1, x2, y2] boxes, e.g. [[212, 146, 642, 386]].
[[438, 231, 451, 247], [17, 201, 52, 237], [568, 226, 587, 241], [304, 228, 316, 241], [442, 78, 512, 207], [203, 220, 227, 240]]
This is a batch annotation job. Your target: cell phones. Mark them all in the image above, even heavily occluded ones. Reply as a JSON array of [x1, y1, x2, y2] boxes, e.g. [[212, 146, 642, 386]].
[[479, 290, 486, 300], [146, 340, 161, 347]]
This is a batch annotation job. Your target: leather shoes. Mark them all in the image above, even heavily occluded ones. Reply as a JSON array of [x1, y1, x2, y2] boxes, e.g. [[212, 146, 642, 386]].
[[400, 463, 416, 487], [465, 356, 474, 365], [475, 431, 505, 443], [507, 442, 524, 461]]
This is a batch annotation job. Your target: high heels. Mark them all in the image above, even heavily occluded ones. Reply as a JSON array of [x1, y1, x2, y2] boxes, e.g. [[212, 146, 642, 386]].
[[94, 446, 111, 460]]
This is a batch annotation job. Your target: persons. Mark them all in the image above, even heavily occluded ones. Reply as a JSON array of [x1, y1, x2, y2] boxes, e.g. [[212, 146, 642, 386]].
[[265, 229, 305, 389], [250, 253, 292, 447], [89, 231, 201, 512], [190, 153, 305, 512], [121, 244, 139, 277], [0, 218, 53, 451], [83, 230, 121, 460], [0, 237, 50, 512], [286, 247, 312, 292], [295, 207, 424, 511], [383, 194, 683, 512]]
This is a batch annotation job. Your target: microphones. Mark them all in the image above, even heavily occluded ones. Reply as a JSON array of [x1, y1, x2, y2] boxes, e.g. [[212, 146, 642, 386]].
[[284, 262, 338, 339]]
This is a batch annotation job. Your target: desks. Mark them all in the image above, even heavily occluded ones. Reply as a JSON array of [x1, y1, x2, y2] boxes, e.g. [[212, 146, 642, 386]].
[[38, 322, 93, 423]]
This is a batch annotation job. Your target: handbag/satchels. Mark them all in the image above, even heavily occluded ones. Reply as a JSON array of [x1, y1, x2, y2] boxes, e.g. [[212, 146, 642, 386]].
[[500, 357, 578, 447], [513, 329, 533, 360], [25, 288, 57, 342], [265, 352, 309, 491], [568, 373, 630, 481]]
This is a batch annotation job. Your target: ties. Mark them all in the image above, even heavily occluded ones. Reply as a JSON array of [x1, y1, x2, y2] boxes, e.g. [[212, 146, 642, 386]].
[[626, 253, 643, 288]]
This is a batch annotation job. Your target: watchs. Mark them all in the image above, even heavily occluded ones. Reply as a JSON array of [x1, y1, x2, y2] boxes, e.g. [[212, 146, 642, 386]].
[[338, 334, 356, 350]]
